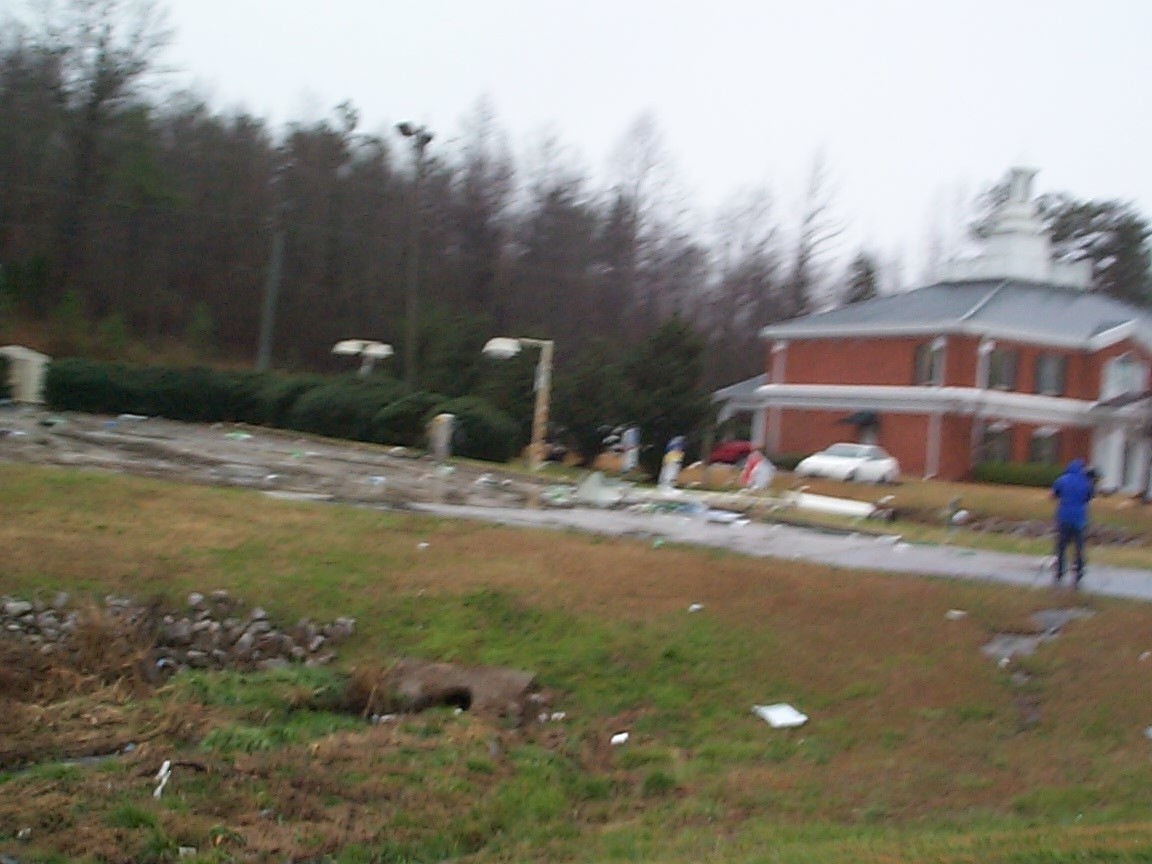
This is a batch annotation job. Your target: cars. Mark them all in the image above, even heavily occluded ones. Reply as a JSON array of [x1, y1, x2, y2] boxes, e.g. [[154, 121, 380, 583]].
[[706, 438, 751, 467], [793, 440, 902, 485]]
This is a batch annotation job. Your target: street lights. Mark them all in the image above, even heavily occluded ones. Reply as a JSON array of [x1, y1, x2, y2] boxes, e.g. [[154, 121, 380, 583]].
[[392, 120, 435, 391]]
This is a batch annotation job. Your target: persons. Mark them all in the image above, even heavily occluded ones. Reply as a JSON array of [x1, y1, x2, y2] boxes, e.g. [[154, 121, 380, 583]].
[[657, 429, 688, 489], [1050, 458, 1095, 594], [621, 422, 642, 472]]
